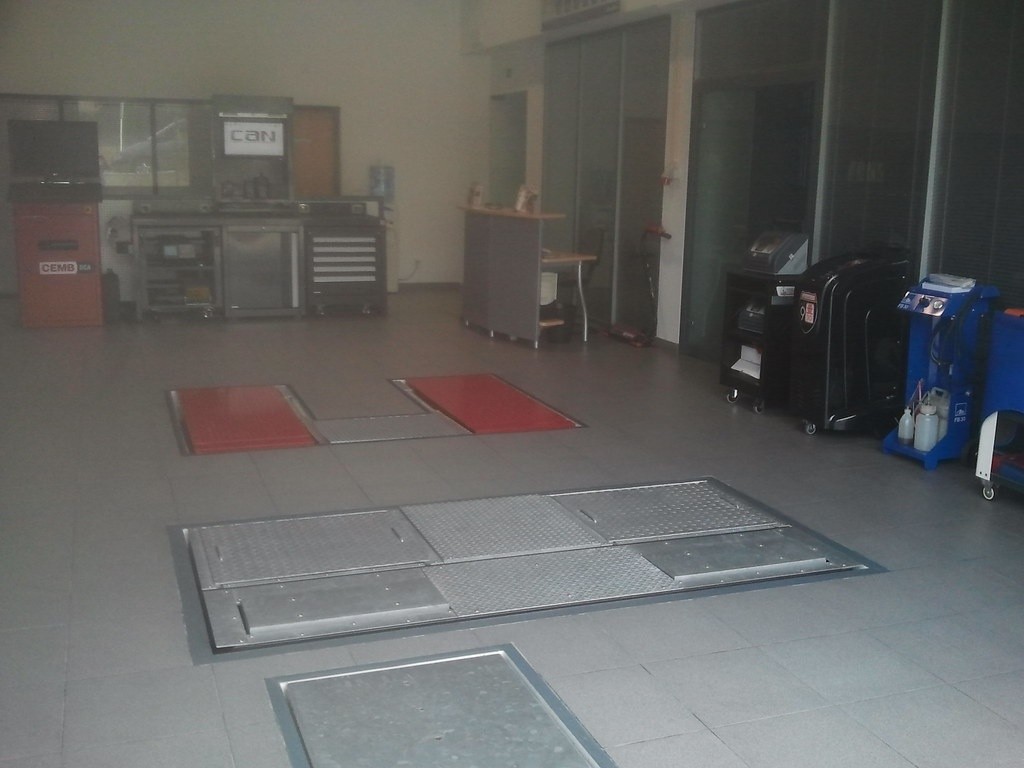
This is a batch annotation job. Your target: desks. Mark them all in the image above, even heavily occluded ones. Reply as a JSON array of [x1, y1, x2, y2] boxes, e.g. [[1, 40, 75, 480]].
[[456, 205, 596, 351]]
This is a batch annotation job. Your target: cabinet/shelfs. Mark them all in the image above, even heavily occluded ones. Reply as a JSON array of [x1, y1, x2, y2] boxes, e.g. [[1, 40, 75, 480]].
[[132, 214, 389, 324]]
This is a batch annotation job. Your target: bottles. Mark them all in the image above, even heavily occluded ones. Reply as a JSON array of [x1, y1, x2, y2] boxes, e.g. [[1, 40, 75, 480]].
[[472, 182, 484, 207], [515, 186, 526, 211], [898, 391, 938, 452], [369, 165, 395, 203], [221, 172, 268, 199]]
